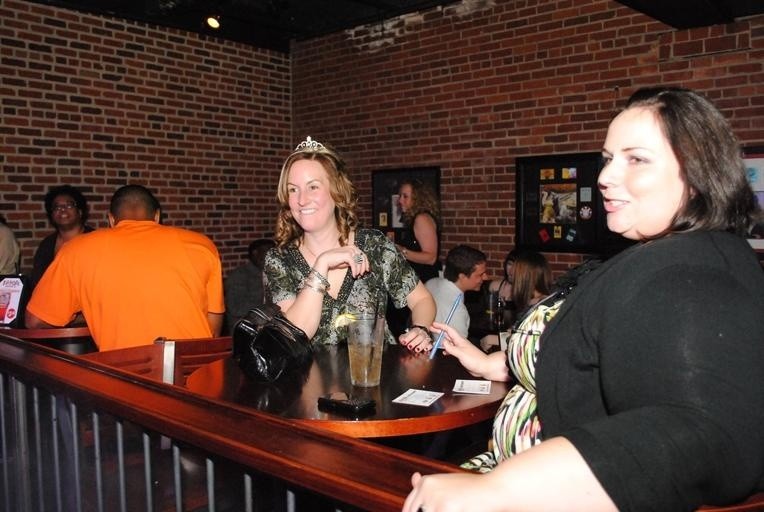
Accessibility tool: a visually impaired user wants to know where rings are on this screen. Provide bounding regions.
[353,255,363,264]
[418,507,423,512]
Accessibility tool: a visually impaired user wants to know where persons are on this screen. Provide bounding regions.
[224,239,276,336]
[486,249,524,311]
[262,135,436,353]
[23,185,226,352]
[401,85,764,512]
[32,185,97,291]
[0,216,21,276]
[394,176,441,283]
[480,252,554,353]
[407,245,488,348]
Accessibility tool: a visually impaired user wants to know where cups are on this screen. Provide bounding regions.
[387,234,394,242]
[346,313,386,388]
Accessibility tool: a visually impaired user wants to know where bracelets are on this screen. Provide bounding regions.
[310,268,330,287]
[410,326,434,341]
[304,280,327,296]
[402,247,407,256]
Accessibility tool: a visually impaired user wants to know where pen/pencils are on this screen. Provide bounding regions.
[428,292,462,360]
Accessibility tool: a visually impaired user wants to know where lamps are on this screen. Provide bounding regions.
[206,13,222,34]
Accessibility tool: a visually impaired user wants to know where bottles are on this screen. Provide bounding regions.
[493,297,505,326]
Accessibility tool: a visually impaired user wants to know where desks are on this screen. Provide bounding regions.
[80,336,508,439]
[0,319,94,355]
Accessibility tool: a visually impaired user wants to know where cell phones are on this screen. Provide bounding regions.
[318,391,377,415]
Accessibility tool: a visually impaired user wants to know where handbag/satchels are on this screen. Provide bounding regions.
[232,303,313,381]
